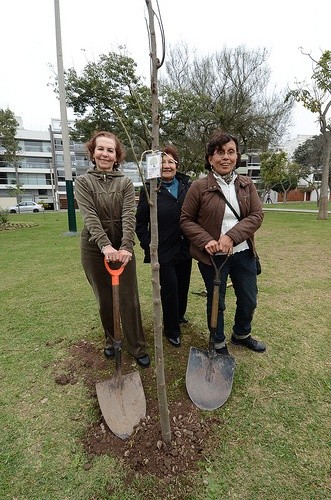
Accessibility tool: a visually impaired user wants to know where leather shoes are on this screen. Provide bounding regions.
[167,336,181,347]
[178,316,188,323]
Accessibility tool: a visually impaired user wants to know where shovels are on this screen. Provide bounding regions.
[186,245,235,411]
[96,252,146,439]
[192,280,233,297]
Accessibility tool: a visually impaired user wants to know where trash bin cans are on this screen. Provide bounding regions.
[43,203,54,210]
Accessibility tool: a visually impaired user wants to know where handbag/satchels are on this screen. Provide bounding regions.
[255,254,261,275]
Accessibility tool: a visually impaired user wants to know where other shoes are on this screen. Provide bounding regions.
[137,352,150,367]
[104,347,116,359]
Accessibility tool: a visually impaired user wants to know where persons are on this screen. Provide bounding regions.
[135,147,195,348]
[180,132,266,355]
[75,132,150,368]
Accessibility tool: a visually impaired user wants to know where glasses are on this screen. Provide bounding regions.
[214,151,237,158]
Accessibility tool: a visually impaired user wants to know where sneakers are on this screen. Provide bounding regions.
[217,344,230,356]
[231,334,266,352]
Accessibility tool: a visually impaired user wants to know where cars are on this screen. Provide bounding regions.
[10,200,45,214]
